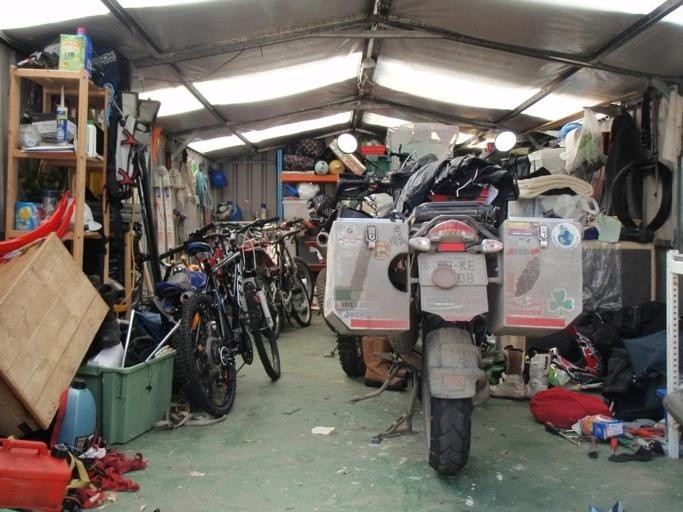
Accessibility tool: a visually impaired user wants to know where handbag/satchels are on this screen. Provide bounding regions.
[556,301,667,362]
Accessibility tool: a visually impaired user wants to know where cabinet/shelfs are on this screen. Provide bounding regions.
[4,61,110,287]
[274,148,363,224]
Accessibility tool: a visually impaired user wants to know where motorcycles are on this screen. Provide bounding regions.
[324,176,585,473]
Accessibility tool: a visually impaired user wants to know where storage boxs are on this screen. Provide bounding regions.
[76,346,178,447]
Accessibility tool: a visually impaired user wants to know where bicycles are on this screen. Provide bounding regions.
[153,217,316,419]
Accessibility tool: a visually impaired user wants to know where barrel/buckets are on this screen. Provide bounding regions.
[58,376,98,453]
[0,439,70,511]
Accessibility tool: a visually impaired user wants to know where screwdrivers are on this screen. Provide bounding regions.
[546,422,578,446]
[611,439,617,456]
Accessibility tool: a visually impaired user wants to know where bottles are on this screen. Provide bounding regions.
[76,27,93,80]
[260,204,267,219]
[86,120,96,157]
[42,190,57,218]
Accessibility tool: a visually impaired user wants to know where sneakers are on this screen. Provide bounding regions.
[488,374,524,399]
[523,379,548,399]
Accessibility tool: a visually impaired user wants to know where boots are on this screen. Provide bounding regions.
[384,338,408,379]
[96,284,120,347]
[361,336,407,390]
